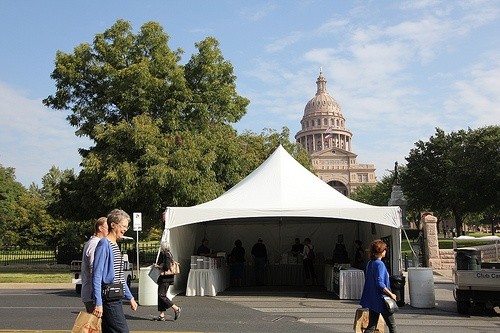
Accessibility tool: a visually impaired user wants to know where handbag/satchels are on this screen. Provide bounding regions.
[101,283,124,301]
[353,308,384,333]
[71,311,102,333]
[149,266,161,284]
[383,296,399,313]
[159,252,180,276]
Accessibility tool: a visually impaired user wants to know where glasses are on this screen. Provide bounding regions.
[118,224,129,229]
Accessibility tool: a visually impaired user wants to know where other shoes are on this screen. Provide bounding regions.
[153,316,165,322]
[174,306,183,321]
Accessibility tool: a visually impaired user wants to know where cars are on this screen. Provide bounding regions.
[54,244,80,264]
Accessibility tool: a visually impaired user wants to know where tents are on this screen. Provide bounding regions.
[163,145,417,306]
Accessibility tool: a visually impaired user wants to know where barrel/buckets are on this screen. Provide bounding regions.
[407,267,435,308]
[138,267,158,306]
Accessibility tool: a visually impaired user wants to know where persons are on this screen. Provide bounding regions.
[93,209,139,333]
[153,240,183,321]
[443,226,456,239]
[196,236,398,333]
[81,217,109,315]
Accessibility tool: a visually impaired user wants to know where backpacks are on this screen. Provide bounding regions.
[306,244,316,264]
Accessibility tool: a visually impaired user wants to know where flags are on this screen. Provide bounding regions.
[325,126,332,140]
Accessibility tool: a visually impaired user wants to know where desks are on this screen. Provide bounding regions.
[325,264,365,300]
[186,266,231,297]
[242,263,324,286]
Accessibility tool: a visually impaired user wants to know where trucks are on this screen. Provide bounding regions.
[451,235,500,317]
